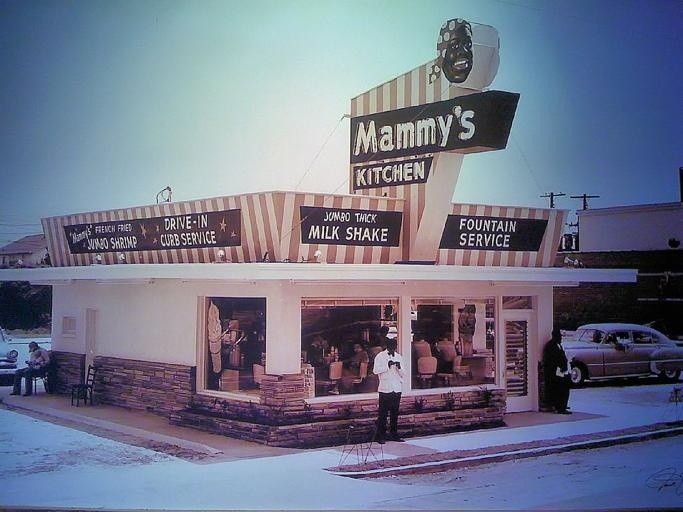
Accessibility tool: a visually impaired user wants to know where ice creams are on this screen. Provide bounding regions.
[208,301,221,373]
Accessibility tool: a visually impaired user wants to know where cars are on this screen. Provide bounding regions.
[0,327,18,385]
[484,315,682,382]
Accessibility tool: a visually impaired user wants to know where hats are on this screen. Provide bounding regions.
[552,330,563,337]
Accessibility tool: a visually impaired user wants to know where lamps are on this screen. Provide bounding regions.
[562,256,585,270]
[213,246,323,263]
[89,252,128,266]
[13,259,22,269]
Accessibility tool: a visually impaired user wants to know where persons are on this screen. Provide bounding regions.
[371,338,405,444]
[234,317,457,395]
[9,340,50,397]
[542,328,573,415]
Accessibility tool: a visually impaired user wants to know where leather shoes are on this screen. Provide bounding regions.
[374,437,385,444]
[557,409,572,414]
[388,435,405,442]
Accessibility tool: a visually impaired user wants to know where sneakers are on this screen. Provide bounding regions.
[10,392,21,396]
[22,391,33,396]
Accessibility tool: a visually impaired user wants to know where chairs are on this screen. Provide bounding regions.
[26,356,54,395]
[71,365,99,407]
[205,332,474,397]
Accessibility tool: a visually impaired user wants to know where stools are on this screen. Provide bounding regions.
[334,419,385,471]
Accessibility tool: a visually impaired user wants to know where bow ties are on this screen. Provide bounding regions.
[388,352,394,357]
